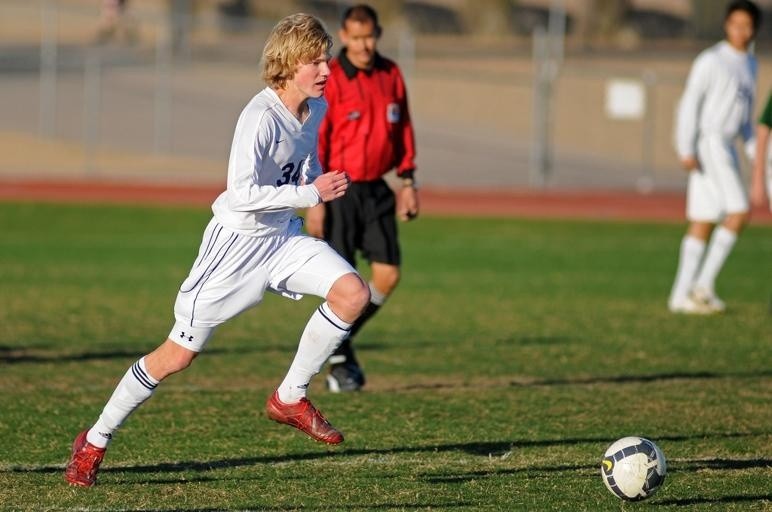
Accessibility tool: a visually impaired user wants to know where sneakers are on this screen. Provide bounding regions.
[264,388,345,445]
[64,428,110,487]
[666,288,726,316]
[322,336,367,395]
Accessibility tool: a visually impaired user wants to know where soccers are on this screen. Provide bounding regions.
[602,437,666,502]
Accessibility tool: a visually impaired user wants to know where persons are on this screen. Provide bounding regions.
[668,1,762,317]
[749,85,772,219]
[66,11,370,491]
[300,3,418,395]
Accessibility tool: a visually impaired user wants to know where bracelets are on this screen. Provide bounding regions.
[402,180,418,191]
